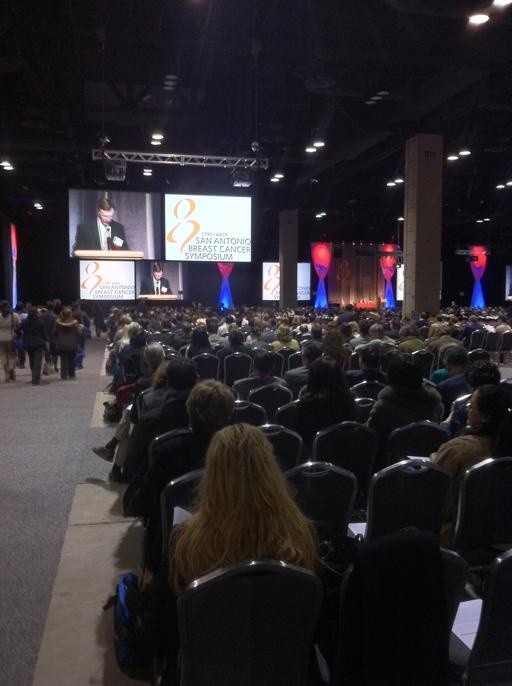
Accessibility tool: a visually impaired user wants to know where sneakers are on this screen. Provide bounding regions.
[109,470,121,481]
[92,446,115,462]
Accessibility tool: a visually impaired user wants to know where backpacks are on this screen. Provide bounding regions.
[112,571,155,682]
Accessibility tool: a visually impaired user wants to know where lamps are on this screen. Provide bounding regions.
[378,242,399,309]
[468,245,488,309]
[216,263,235,310]
[309,242,333,309]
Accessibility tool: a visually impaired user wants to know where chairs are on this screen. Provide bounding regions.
[324,546,467,684]
[154,560,323,685]
[467,328,512,368]
[450,549,512,686]
[136,340,512,557]
[418,325,429,340]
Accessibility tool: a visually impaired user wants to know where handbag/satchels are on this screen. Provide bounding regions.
[70,348,85,368]
[43,352,55,376]
[103,402,122,422]
[110,367,124,392]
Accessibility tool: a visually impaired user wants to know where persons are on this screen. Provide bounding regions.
[73,198,128,252]
[140,263,172,295]
